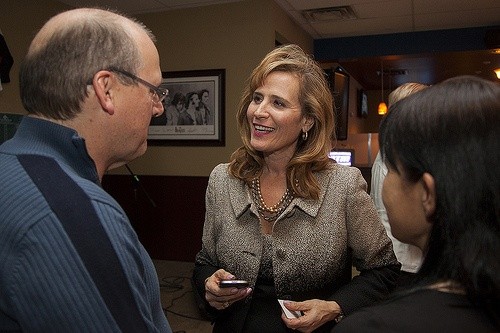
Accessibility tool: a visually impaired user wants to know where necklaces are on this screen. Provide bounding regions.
[250,164,302,223]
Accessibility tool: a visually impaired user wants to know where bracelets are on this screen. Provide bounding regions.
[334,310,344,323]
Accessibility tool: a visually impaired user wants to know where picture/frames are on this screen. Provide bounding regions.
[146,68,226,147]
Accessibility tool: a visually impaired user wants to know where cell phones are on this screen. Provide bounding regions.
[218,280,249,289]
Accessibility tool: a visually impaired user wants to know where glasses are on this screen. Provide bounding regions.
[86,64,170,105]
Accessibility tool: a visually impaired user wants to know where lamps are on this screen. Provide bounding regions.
[378,58,388,115]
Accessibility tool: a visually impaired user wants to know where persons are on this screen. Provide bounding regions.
[0,9,174,333]
[328,75,500,332]
[189,45,399,333]
[370,81,444,279]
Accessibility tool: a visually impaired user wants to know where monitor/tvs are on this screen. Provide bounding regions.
[358,89,368,118]
[328,148,355,168]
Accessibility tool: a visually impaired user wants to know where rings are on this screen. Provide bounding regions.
[224,302,229,307]
[292,328,295,330]
